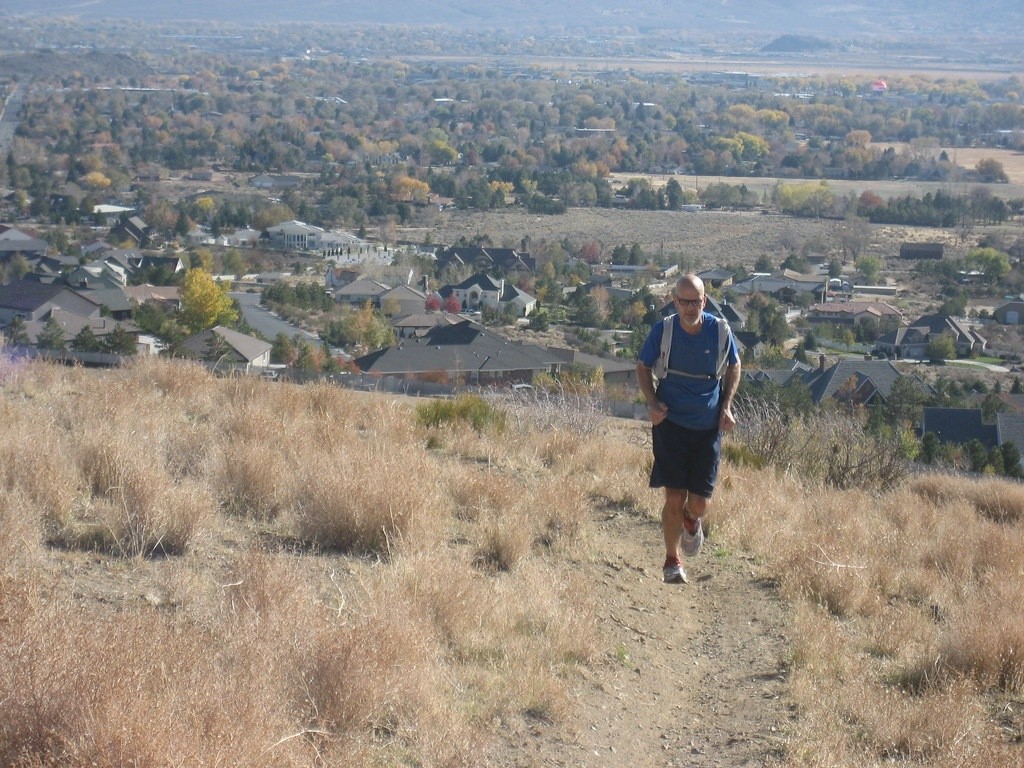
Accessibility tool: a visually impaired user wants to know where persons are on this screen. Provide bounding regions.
[637,275,741,584]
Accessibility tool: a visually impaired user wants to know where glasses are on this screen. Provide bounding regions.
[677,298,703,307]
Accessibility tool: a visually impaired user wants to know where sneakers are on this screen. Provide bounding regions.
[682,500,706,557]
[663,563,688,584]
